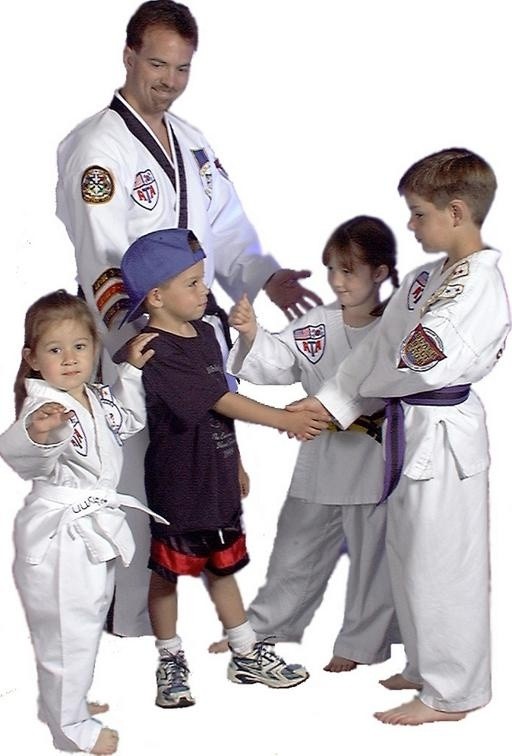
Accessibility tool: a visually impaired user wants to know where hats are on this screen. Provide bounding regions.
[119,228,207,331]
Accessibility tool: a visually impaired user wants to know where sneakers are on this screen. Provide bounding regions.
[228,636,309,688]
[155,647,195,708]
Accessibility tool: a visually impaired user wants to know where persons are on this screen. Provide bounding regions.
[207,214,406,672]
[1,287,162,756]
[118,225,332,711]
[279,146,511,731]
[54,0,324,641]
